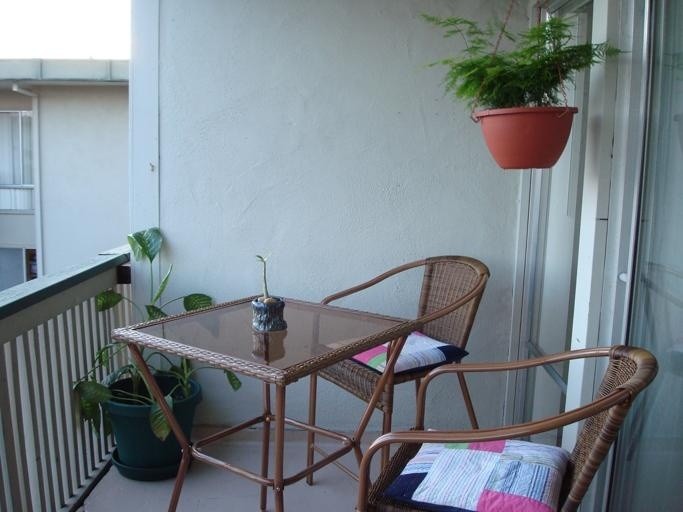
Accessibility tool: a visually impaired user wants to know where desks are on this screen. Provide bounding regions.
[112,290,419,512]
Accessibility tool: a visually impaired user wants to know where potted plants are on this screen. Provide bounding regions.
[70,226,238,483]
[419,2,629,168]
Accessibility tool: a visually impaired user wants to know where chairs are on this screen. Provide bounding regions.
[305,256,490,492]
[357,346,660,512]
